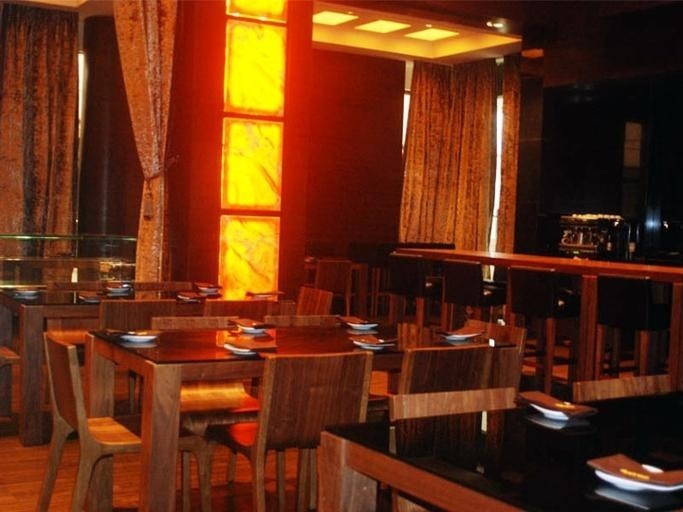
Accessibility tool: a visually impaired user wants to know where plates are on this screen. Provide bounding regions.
[353,339,395,350]
[224,343,269,356]
[347,323,378,330]
[14,291,40,296]
[177,287,219,303]
[441,333,481,340]
[78,287,130,304]
[595,463,683,494]
[238,325,266,334]
[529,399,580,421]
[121,336,158,343]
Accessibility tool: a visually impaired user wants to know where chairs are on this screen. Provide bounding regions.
[572,375,671,404]
[206,351,374,512]
[1,251,526,480]
[35,332,211,511]
[389,386,515,421]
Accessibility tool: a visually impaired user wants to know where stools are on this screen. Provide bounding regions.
[508,265,584,392]
[592,272,670,382]
